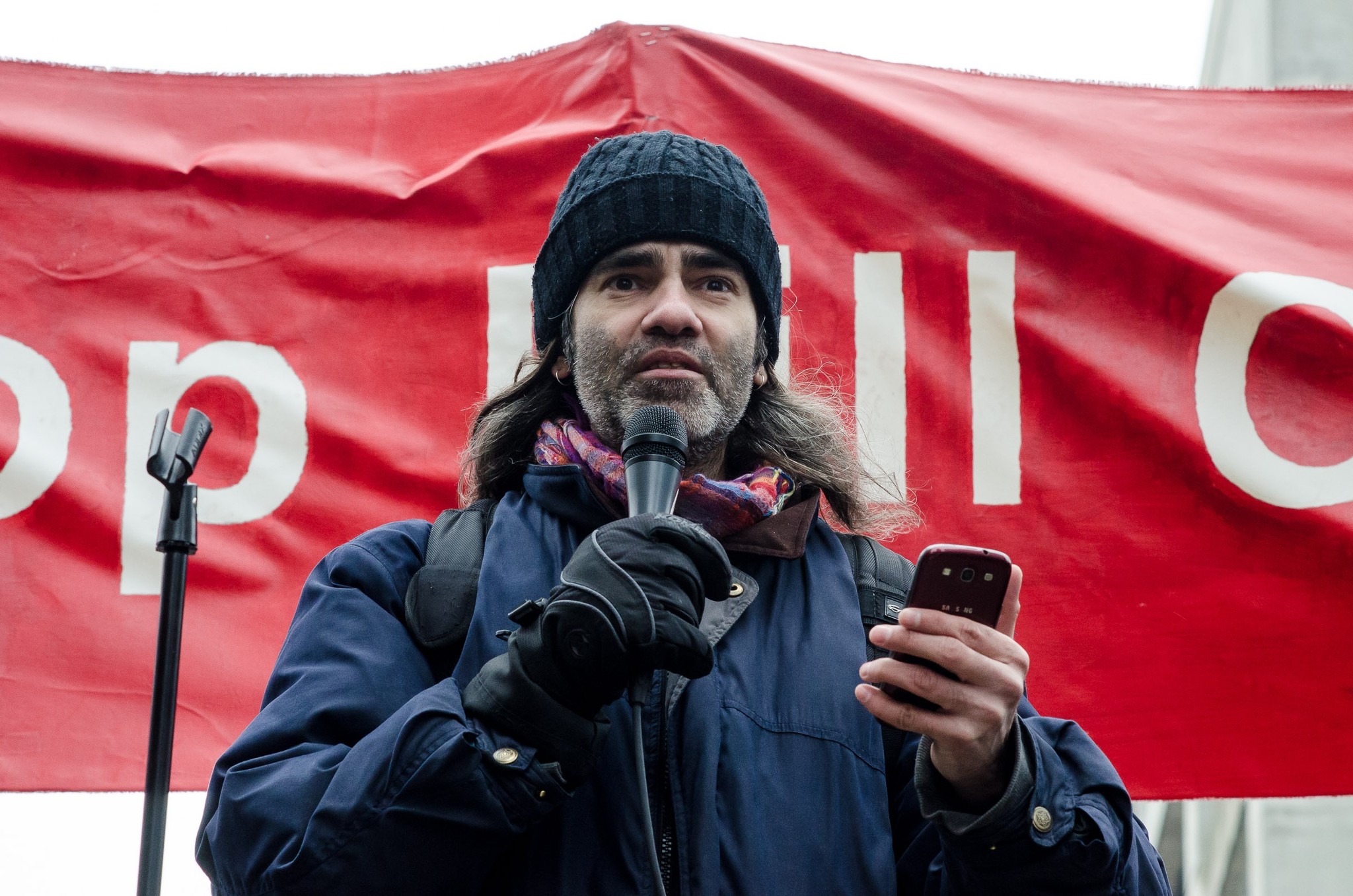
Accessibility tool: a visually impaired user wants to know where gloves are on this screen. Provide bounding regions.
[511,512,735,718]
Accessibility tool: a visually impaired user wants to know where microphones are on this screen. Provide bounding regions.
[619,404,690,710]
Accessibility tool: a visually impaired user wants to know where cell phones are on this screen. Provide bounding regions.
[873,544,1012,734]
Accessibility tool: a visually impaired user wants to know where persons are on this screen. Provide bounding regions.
[195,132,1173,896]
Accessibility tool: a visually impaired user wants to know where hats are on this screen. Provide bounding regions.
[533,130,780,371]
